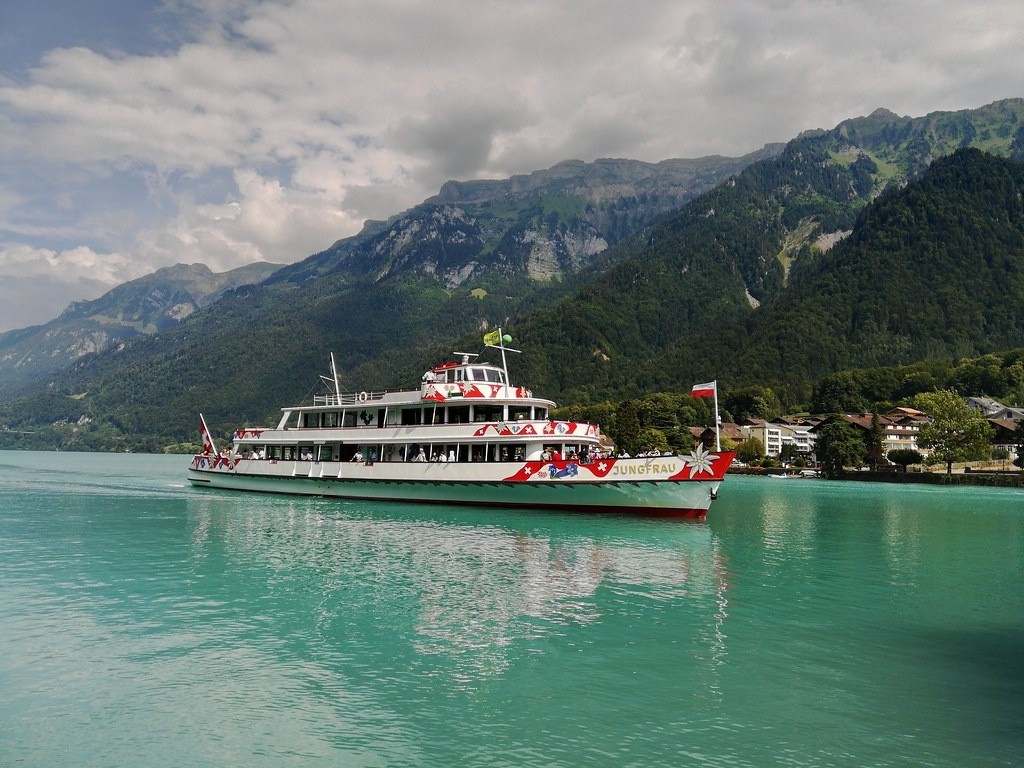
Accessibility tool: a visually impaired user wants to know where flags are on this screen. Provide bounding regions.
[690,382,714,398]
[483,330,500,346]
[199,416,213,452]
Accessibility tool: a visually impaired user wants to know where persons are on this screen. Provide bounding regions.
[577,446,676,464]
[349,450,364,462]
[371,448,377,461]
[413,448,482,463]
[221,446,264,460]
[233,428,238,439]
[288,451,313,461]
[540,448,562,462]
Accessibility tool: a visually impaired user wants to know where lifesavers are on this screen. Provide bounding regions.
[359,392,368,403]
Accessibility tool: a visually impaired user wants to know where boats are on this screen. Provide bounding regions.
[767,472,801,478]
[186,328,738,520]
